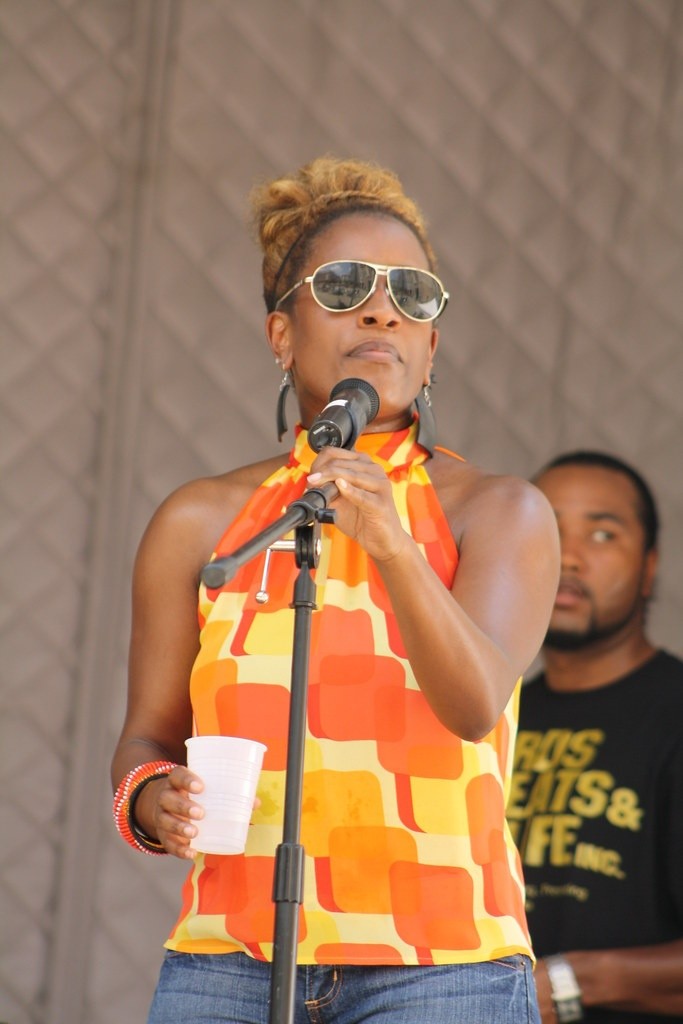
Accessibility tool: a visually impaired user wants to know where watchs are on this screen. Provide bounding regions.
[543,952,583,1024]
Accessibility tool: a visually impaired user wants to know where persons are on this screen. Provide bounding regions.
[111,156,561,1022]
[512,449,683,1023]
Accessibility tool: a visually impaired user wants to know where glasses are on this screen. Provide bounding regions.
[270,260,450,323]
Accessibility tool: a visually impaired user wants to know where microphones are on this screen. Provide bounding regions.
[308,378,380,455]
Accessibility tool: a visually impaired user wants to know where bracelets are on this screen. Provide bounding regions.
[113,761,183,861]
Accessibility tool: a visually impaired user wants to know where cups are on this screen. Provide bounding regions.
[183,735,268,856]
